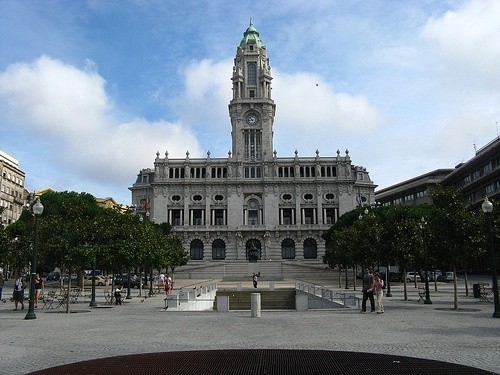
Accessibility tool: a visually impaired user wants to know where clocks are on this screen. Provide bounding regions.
[247,111,260,126]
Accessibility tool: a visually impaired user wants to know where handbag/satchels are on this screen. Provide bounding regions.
[379,279,386,290]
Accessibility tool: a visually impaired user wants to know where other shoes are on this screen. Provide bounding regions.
[370,310,376,313]
[35,306,37,308]
[21,308,24,310]
[360,309,367,313]
[13,309,18,311]
[376,311,384,315]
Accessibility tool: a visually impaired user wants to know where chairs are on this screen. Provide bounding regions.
[103,289,126,307]
[417,285,429,301]
[478,281,495,304]
[42,288,81,309]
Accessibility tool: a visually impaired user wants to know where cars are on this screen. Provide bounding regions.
[356,269,458,283]
[20,273,30,282]
[46,271,60,281]
[58,269,175,290]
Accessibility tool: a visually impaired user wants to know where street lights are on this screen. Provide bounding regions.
[482,196,500,318]
[420,216,433,304]
[139,210,156,289]
[24,198,44,320]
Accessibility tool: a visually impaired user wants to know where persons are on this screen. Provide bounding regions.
[34,273,44,308]
[360,266,375,313]
[160,272,173,295]
[12,274,25,311]
[0,273,4,300]
[105,274,109,286]
[253,273,260,288]
[369,272,384,314]
